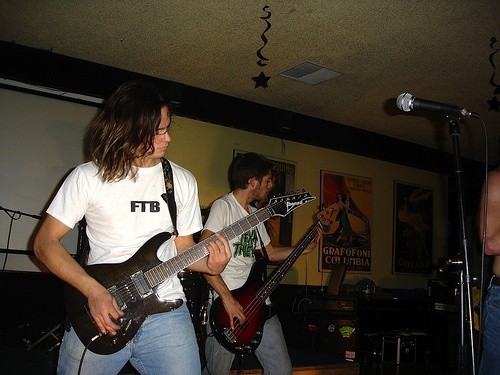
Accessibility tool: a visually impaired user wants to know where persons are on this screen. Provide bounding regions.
[196,151,325,375]
[30,80,233,375]
[476,165,500,375]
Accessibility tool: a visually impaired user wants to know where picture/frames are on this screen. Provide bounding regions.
[319,169,372,275]
[391,179,437,278]
[233,149,299,250]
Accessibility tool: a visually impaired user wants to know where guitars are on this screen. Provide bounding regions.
[68,188,318,355]
[209,202,344,355]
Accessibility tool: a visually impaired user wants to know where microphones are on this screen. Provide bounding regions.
[396,93,479,118]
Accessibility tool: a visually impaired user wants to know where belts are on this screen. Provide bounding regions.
[492,276,500,286]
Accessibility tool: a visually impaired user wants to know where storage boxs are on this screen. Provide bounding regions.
[380,334,417,366]
